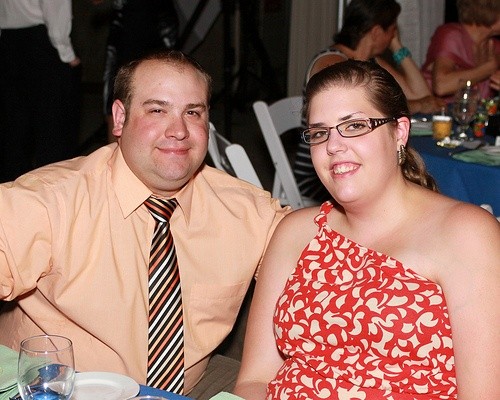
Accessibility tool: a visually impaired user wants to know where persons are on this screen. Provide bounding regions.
[0,0,83,171]
[233,0,500,400]
[0,51,290,400]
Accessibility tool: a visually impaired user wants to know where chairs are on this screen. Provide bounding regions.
[207,121,263,197]
[253,95,319,212]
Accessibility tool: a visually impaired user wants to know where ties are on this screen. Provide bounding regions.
[142,196,186,395]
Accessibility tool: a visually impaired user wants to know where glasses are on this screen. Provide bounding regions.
[300,116,398,145]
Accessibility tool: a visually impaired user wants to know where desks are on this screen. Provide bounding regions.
[409,112,500,217]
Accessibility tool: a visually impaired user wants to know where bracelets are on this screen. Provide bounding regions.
[393,47,411,63]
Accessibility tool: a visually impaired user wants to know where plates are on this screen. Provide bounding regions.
[68,372,140,400]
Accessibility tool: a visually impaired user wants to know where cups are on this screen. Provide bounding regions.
[431,116,454,142]
[17,334,76,400]
